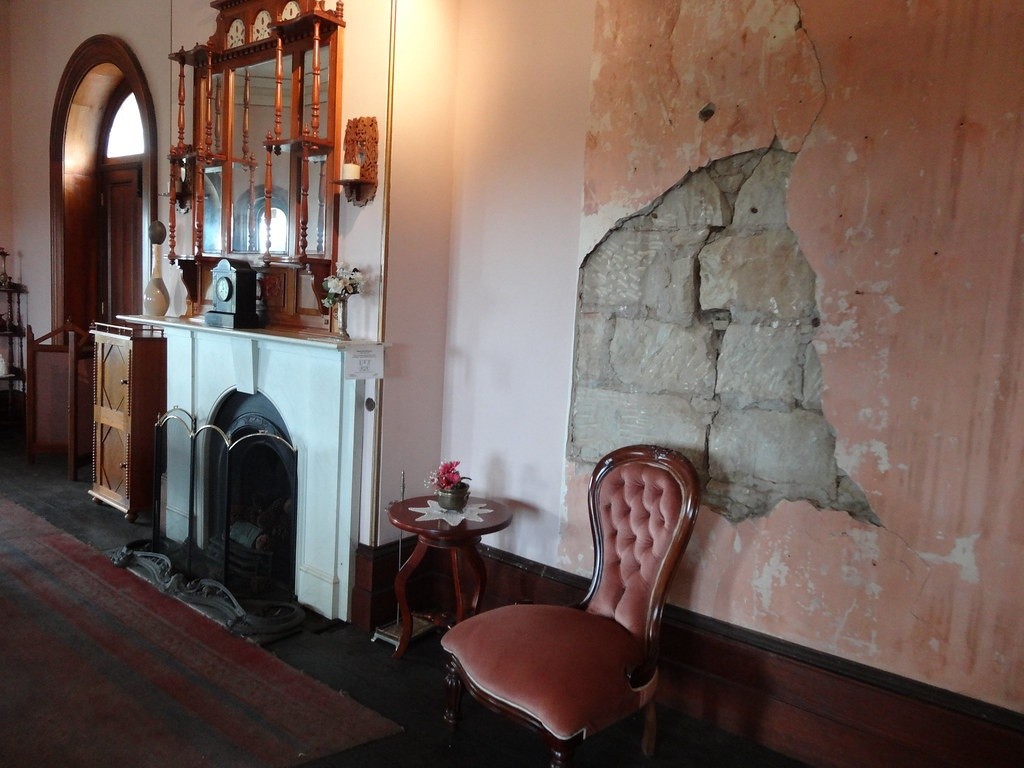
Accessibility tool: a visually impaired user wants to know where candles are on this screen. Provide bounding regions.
[341,160,360,180]
[167,180,181,193]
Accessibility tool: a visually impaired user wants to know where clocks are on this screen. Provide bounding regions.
[204,257,258,331]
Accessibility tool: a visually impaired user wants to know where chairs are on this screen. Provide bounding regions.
[438,443,703,768]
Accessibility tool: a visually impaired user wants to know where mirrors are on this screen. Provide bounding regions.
[204,46,326,258]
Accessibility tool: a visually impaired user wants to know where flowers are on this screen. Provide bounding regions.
[321,260,370,309]
[422,458,472,496]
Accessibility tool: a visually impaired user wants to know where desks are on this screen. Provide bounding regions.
[0,496,408,768]
[387,496,512,660]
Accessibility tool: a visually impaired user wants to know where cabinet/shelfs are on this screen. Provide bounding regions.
[0,286,26,440]
[87,320,168,523]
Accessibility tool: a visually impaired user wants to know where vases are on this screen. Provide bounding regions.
[436,483,471,514]
[337,301,351,341]
